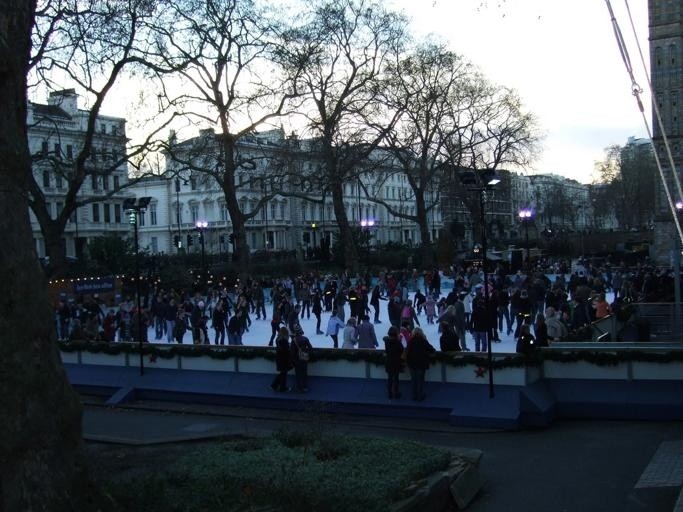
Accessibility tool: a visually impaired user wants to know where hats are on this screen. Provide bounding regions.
[593,294,601,302]
[387,326,398,336]
[537,313,546,320]
[294,325,304,334]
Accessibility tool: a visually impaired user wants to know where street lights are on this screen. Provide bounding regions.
[172,176,189,249]
[196,222,208,266]
[459,176,502,400]
[360,219,375,255]
[471,244,479,260]
[310,222,318,257]
[121,194,153,379]
[517,209,534,273]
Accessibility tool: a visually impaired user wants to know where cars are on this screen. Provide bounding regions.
[39,254,78,269]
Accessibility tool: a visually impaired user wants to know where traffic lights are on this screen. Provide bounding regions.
[228,233,233,244]
[186,234,194,248]
[172,234,178,248]
[220,235,225,243]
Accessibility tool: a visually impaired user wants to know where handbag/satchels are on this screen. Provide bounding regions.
[299,351,309,360]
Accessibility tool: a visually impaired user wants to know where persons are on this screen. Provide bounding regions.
[42,225,683,401]
